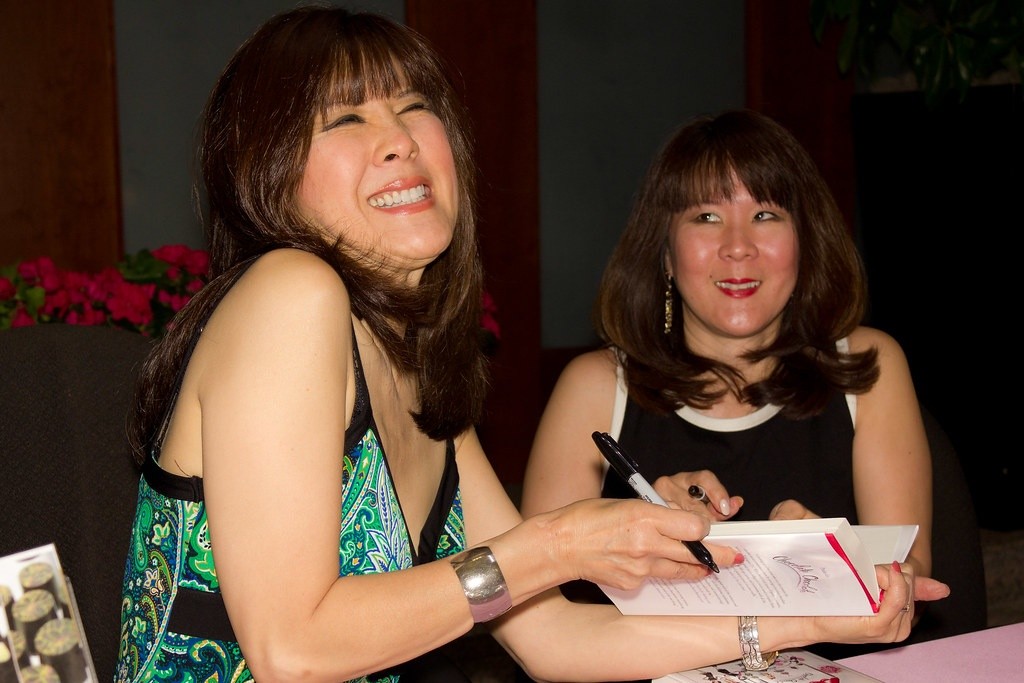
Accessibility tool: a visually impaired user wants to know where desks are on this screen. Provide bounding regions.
[834,620,1024,682]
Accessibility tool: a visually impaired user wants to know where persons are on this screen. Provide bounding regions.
[114,5,950,683]
[517,108,934,662]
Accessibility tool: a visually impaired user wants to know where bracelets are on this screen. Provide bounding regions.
[448,545,513,625]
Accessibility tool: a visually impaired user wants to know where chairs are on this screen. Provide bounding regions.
[914,406,988,644]
[0,321,160,683]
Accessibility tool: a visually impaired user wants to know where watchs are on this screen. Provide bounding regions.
[738,616,779,672]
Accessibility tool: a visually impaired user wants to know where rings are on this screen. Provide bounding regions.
[902,604,911,613]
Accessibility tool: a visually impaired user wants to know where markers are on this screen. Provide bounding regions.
[686,480,712,507]
[591,430,721,577]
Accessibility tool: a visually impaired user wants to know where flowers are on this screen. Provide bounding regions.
[0,241,505,338]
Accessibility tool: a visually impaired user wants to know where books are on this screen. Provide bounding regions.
[597,518,920,616]
